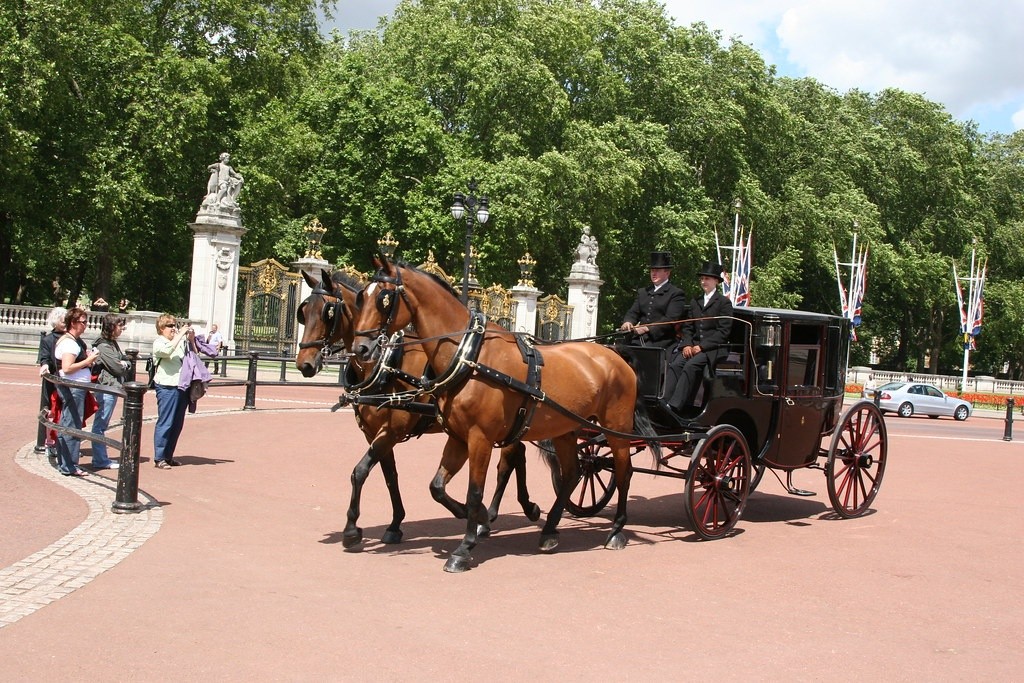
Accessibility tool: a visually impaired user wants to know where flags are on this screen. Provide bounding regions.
[717,238,751,306]
[958,277,983,350]
[839,267,866,341]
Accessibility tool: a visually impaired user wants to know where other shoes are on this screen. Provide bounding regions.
[79,452,83,457]
[155,458,182,469]
[61,466,89,476]
[47,447,57,457]
[656,396,671,411]
[93,460,119,469]
[34,446,45,453]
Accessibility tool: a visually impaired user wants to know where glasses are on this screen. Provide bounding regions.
[73,320,88,324]
[164,324,176,327]
[116,323,125,326]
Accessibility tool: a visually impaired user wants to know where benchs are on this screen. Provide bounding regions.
[611,336,779,407]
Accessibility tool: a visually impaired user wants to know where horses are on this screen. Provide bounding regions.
[293,248,664,574]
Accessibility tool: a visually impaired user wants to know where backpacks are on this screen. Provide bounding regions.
[145,356,163,390]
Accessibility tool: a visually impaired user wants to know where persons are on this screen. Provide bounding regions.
[53,308,100,476]
[908,377,913,382]
[203,324,222,374]
[118,298,128,326]
[91,313,132,468]
[619,251,686,348]
[863,375,876,399]
[39,307,69,456]
[153,313,199,469]
[661,261,733,411]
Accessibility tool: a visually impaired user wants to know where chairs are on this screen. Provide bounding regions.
[917,387,922,393]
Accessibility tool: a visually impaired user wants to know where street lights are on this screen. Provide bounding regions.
[451,193,491,307]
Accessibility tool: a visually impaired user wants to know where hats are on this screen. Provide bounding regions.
[696,261,724,284]
[647,252,674,268]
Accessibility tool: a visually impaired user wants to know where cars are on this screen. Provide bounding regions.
[875,382,974,420]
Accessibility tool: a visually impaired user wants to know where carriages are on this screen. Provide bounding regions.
[295,252,887,571]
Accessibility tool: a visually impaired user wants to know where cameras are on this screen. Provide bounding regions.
[91,347,99,355]
[185,328,194,335]
